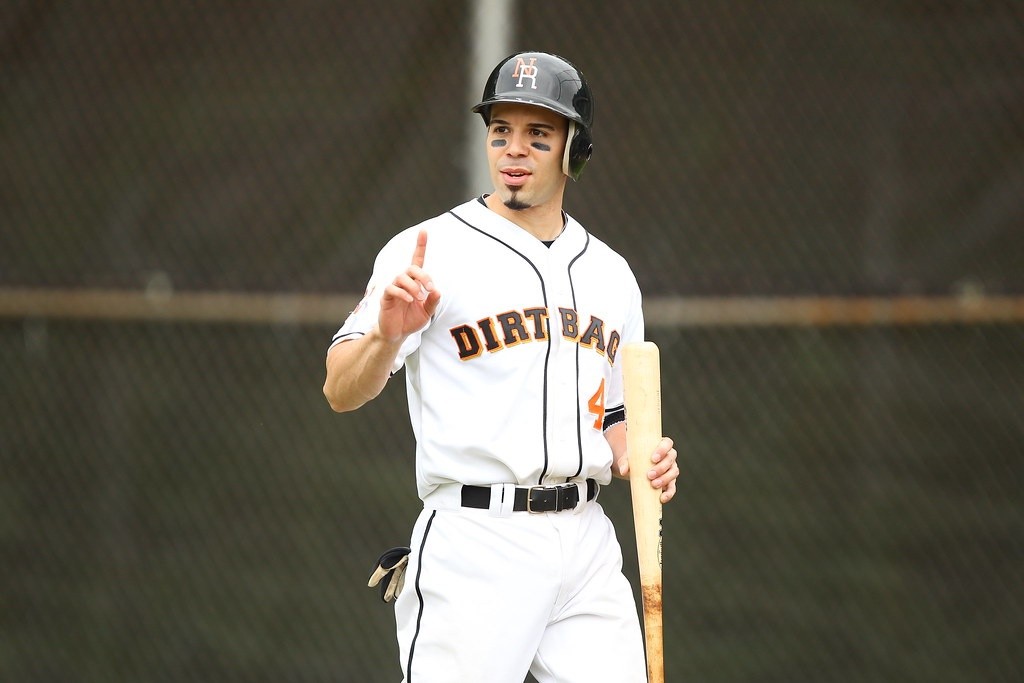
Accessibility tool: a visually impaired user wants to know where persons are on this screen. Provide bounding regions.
[323,50,679,683]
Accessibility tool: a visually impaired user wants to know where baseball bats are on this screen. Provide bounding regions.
[619,340,680,683]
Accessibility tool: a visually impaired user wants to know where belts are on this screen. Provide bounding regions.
[461,478,597,514]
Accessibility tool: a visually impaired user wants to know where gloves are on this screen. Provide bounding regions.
[366,546,411,604]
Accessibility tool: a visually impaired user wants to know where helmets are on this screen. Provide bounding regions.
[470,50,595,183]
